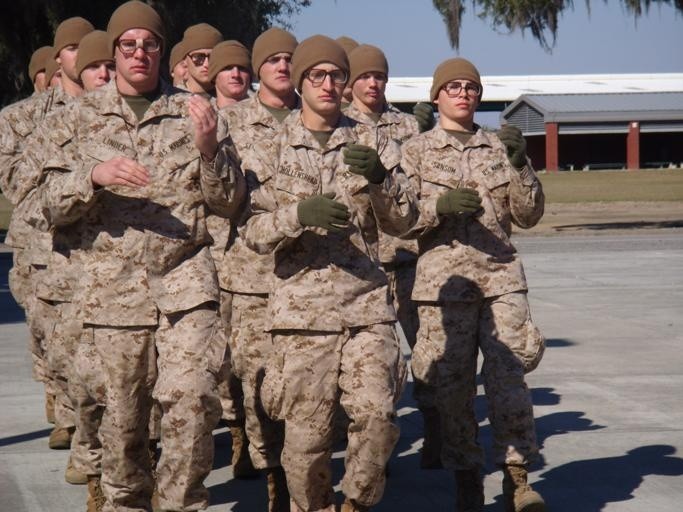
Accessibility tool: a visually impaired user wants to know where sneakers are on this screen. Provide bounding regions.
[47,404,57,424]
[86,476,106,512]
[454,470,484,511]
[65,461,89,484]
[49,427,70,449]
[502,464,545,511]
[232,424,261,479]
[420,446,443,470]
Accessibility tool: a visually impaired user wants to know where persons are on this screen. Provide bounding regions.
[398,54,553,512]
[30,33,118,488]
[330,36,364,58]
[204,38,253,110]
[167,41,194,90]
[41,0,249,512]
[174,19,230,93]
[212,26,332,481]
[5,16,100,453]
[345,46,448,467]
[0,45,50,247]
[242,35,422,512]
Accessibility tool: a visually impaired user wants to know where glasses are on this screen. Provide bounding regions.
[115,38,161,54]
[187,52,210,66]
[304,69,348,85]
[441,82,483,99]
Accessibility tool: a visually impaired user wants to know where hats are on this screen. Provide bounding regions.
[430,58,482,102]
[46,51,61,89]
[29,46,53,82]
[170,41,184,72]
[107,1,164,59]
[348,44,388,88]
[75,30,115,80]
[335,37,359,53]
[54,17,94,59]
[252,28,300,80]
[208,40,252,82]
[290,35,350,95]
[184,23,223,58]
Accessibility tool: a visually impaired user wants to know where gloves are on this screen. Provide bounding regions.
[436,189,484,215]
[343,144,386,183]
[298,190,351,235]
[414,102,436,132]
[497,126,528,168]
